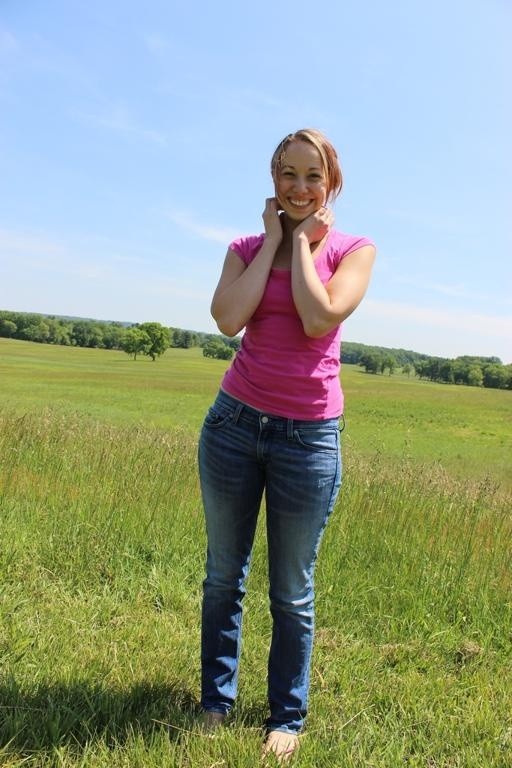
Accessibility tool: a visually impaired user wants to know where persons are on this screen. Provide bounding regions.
[199,128,376,755]
